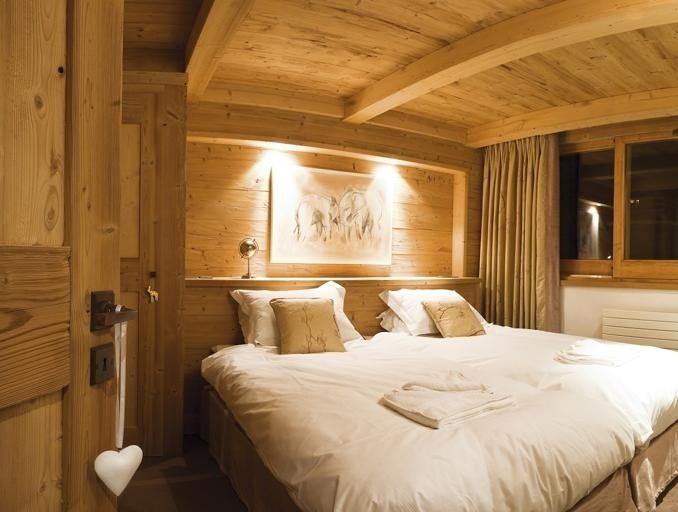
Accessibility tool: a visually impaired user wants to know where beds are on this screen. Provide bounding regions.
[200,323,678,512]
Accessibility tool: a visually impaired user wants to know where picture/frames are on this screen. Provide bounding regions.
[270,165,393,266]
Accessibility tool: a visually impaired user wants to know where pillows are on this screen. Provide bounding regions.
[230,279,490,355]
